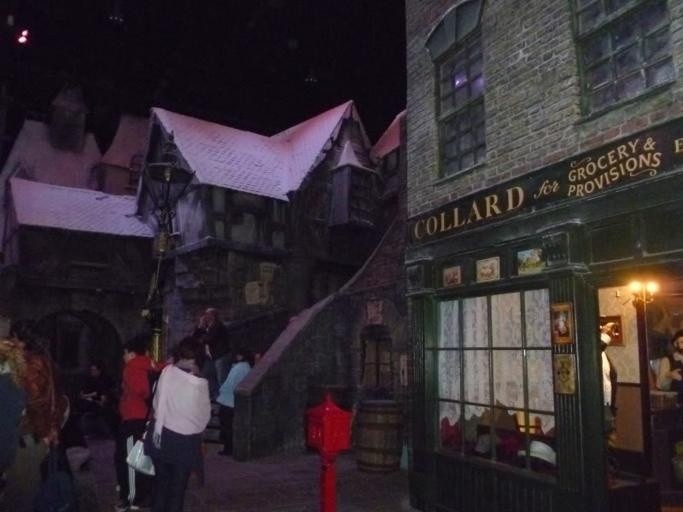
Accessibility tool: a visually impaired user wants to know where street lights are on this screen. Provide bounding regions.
[132,120,196,375]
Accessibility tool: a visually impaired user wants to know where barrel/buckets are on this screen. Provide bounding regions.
[354,398,403,475]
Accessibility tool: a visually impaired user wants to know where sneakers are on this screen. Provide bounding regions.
[114,502,140,511]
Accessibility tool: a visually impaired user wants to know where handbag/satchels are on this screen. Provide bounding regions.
[126,425,160,477]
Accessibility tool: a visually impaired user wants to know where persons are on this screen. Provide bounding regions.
[601,331,619,489]
[656,329,683,409]
[2,306,253,511]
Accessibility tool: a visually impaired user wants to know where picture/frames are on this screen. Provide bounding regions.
[599,314,624,348]
[549,302,575,345]
